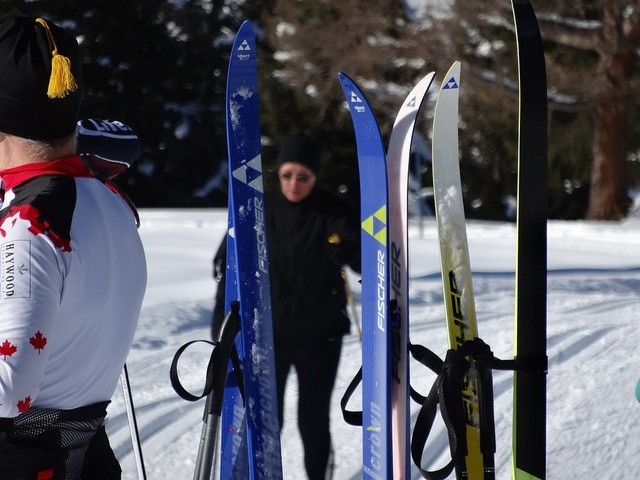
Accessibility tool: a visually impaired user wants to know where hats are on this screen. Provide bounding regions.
[0,17,82,140]
[278,134,319,176]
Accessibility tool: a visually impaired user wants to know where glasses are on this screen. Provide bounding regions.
[279,171,311,185]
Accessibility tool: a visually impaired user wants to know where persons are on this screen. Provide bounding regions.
[212,133,362,479]
[0,17,147,480]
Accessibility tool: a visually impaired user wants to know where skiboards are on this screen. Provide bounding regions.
[220,19,283,480]
[431,0,548,480]
[337,71,436,480]
